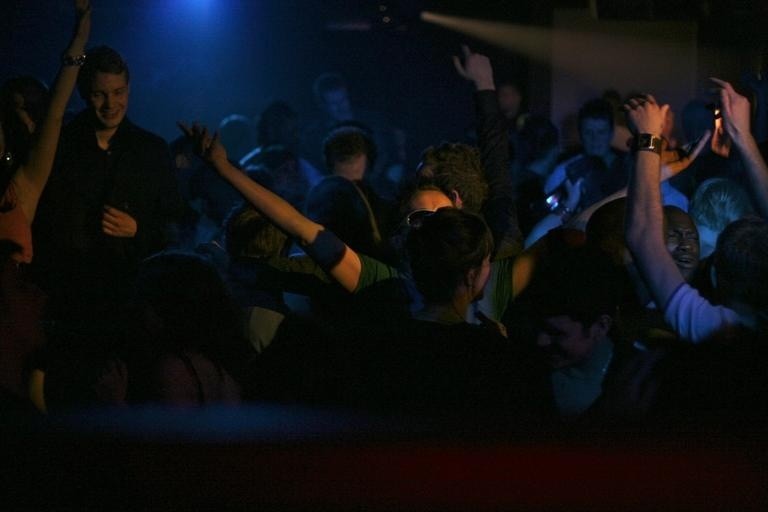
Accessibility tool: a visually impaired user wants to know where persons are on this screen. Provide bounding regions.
[1,1,768,509]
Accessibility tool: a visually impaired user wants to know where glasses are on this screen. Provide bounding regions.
[396,208,450,228]
